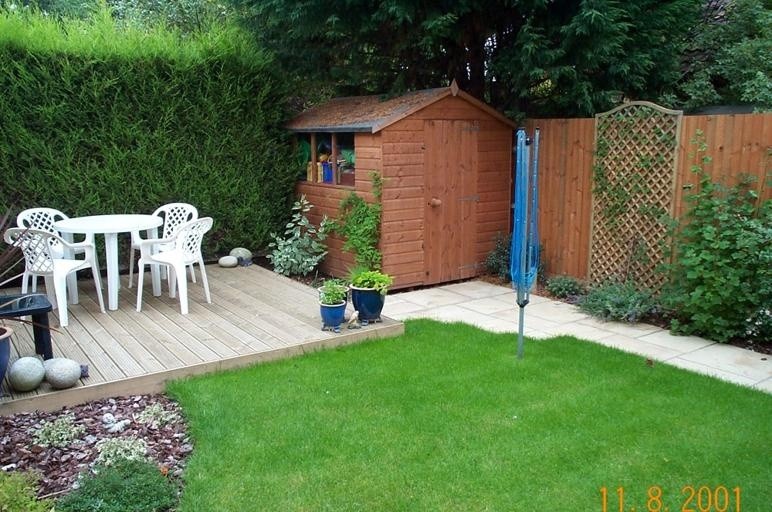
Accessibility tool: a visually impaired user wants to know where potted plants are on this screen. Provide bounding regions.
[317,279,349,333]
[344,271,395,324]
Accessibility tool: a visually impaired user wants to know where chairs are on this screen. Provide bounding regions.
[3,228,106,329]
[17,208,104,306]
[136,217,213,315]
[128,203,198,287]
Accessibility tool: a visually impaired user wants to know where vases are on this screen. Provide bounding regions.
[0,326,13,385]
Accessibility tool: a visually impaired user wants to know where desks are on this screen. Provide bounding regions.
[55,214,163,310]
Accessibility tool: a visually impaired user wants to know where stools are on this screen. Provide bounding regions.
[0,293,53,361]
